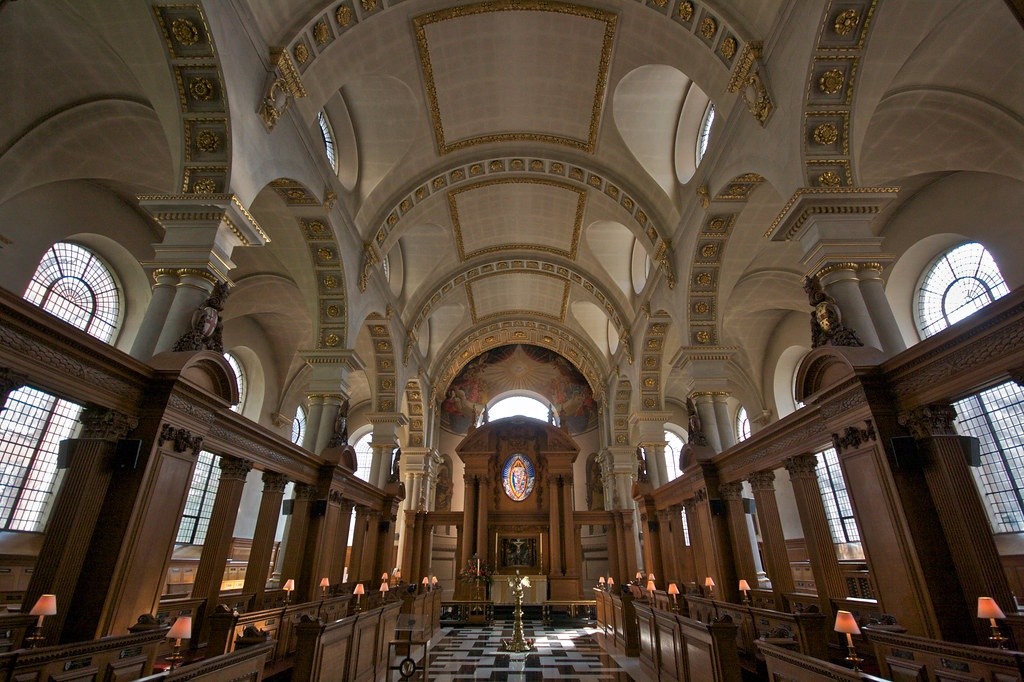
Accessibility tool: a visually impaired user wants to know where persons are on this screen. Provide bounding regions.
[435,464,454,513]
[585,462,606,512]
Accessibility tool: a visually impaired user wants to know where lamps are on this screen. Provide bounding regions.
[283,579,294,606]
[432,576,438,589]
[978,597,1008,650]
[25,594,57,650]
[668,583,681,614]
[353,584,364,614]
[739,580,752,605]
[599,572,656,597]
[394,572,401,584]
[164,617,192,671]
[320,577,329,599]
[833,610,865,671]
[705,577,715,600]
[379,582,389,606]
[381,573,388,582]
[422,577,428,593]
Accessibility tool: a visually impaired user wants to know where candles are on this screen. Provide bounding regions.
[477,559,480,576]
[495,532,498,555]
[540,533,542,554]
